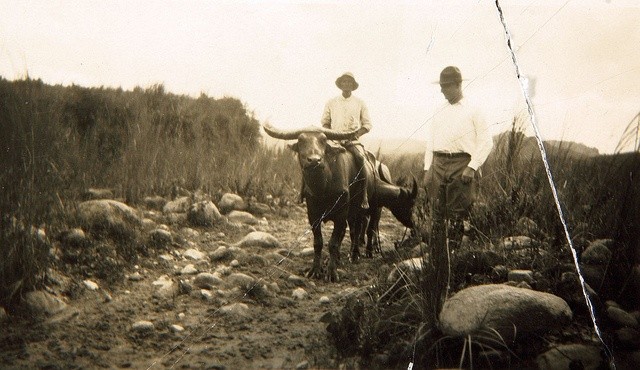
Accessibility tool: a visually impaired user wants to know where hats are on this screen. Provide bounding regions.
[336,70,359,89]
[430,66,470,84]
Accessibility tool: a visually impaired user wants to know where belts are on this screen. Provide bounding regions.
[435,151,470,158]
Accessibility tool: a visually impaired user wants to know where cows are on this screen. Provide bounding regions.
[263,123,377,285]
[355,159,418,259]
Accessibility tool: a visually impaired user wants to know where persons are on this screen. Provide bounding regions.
[423,66,494,218]
[321,72,372,209]
